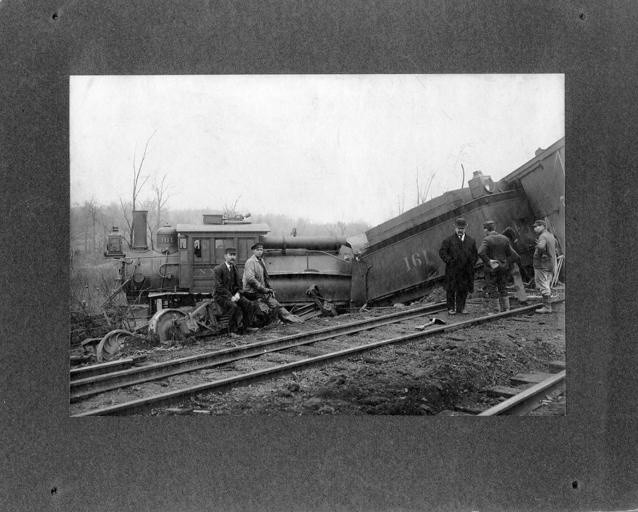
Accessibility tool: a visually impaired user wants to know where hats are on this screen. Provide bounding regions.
[251,242,264,250]
[453,218,468,227]
[483,221,495,226]
[225,248,237,254]
[529,220,546,227]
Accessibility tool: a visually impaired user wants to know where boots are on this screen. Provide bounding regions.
[535,295,552,314]
[488,297,510,316]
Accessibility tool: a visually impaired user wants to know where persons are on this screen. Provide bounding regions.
[214,242,298,338]
[528,220,558,314]
[439,218,530,316]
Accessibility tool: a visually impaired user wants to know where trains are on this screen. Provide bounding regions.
[104,136,565,305]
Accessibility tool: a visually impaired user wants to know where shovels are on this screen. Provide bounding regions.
[415,318,446,331]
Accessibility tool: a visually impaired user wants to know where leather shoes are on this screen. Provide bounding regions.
[448,310,470,314]
[244,328,259,334]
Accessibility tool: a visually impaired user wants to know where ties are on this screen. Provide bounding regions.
[230,263,233,273]
[459,235,462,240]
